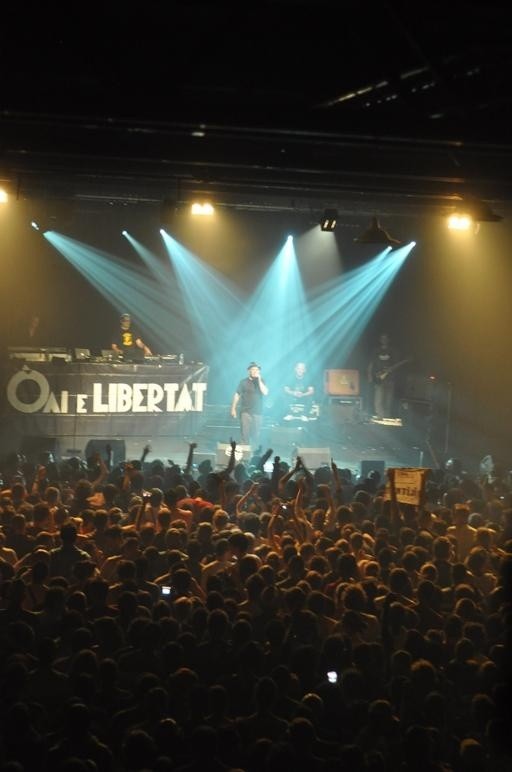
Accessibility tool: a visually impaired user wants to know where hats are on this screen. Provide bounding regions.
[248,362,260,370]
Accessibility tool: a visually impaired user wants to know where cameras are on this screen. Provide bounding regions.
[160,584,173,596]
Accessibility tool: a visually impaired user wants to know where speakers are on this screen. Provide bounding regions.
[291,448,331,469]
[85,439,125,462]
[23,435,60,459]
[216,444,251,466]
[327,369,361,396]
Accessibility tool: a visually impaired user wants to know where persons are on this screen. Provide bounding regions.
[112,313,154,356]
[1,439,510,771]
[366,333,409,421]
[281,361,315,416]
[11,312,48,346]
[229,360,269,443]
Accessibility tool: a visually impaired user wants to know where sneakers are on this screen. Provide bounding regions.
[377,415,382,420]
